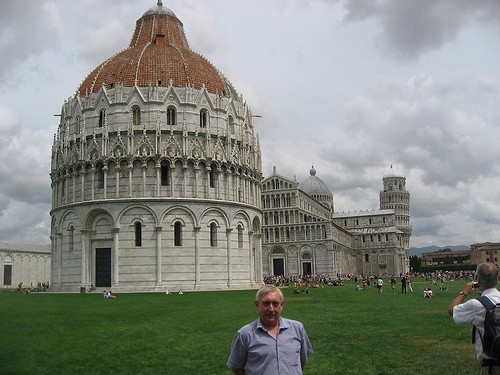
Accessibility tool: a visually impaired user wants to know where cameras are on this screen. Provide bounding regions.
[472,281,480,288]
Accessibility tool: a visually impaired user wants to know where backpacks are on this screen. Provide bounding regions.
[472,295,500,358]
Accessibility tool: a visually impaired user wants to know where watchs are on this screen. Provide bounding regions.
[460,291,467,297]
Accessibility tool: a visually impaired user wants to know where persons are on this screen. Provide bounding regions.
[263,269,482,298]
[447,262,500,375]
[1,281,119,299]
[226,285,314,375]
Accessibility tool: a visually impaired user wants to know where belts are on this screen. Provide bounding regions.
[480,359,497,368]
